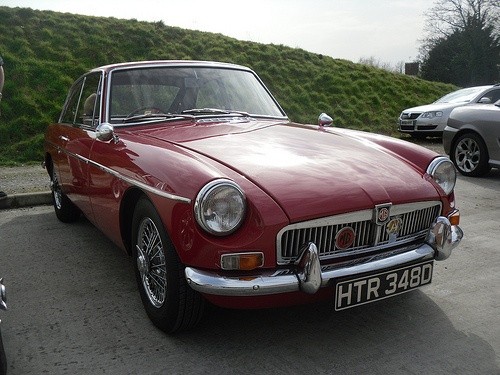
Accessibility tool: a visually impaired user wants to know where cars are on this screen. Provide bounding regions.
[42,58,463,335]
[396,83,500,134]
[443,83,500,174]
